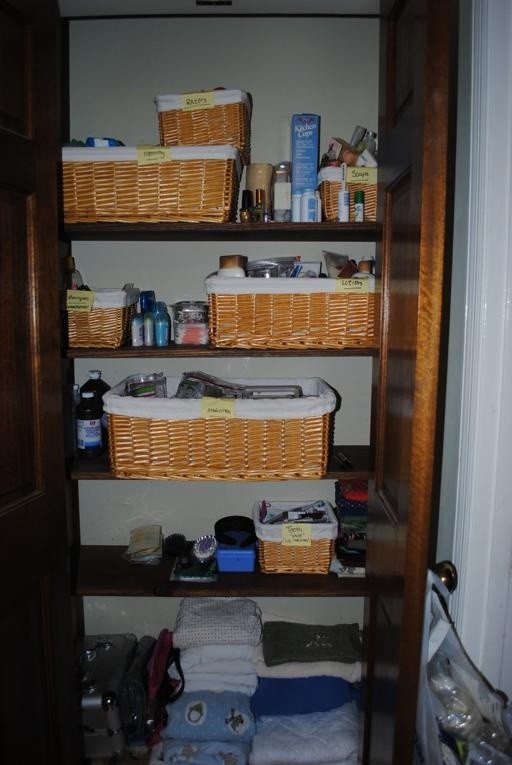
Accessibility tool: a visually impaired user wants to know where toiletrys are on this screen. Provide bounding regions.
[130,290,170,347]
[236,184,366,224]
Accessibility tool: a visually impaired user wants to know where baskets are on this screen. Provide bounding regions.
[153,89,251,165]
[203,268,380,351]
[102,372,339,480]
[65,287,138,349]
[316,165,378,222]
[252,497,339,576]
[61,144,244,225]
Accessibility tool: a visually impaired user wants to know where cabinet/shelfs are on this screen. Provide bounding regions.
[1,0,463,764]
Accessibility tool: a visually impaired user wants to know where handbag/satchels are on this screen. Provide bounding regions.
[118,626,177,750]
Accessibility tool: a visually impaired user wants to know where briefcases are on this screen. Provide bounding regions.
[79,632,139,761]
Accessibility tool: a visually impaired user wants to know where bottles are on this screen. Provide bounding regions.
[360,129,378,152]
[349,261,374,279]
[131,293,169,347]
[354,189,364,222]
[289,186,325,225]
[72,368,107,452]
[215,256,245,278]
